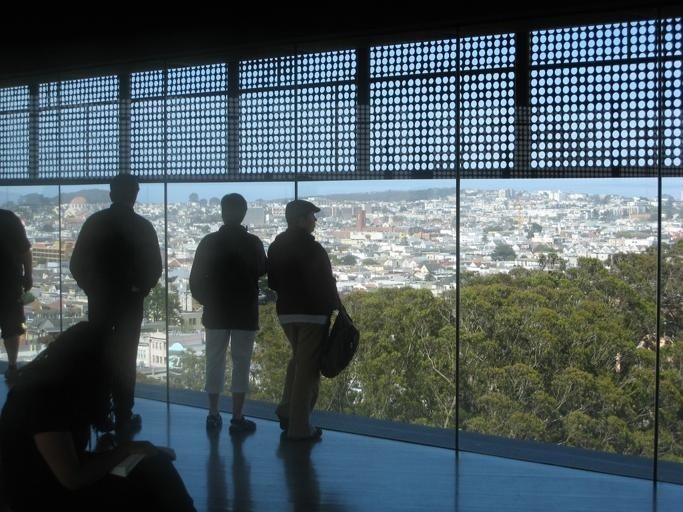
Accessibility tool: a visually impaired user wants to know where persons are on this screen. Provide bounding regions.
[0,205,33,379]
[188,193,269,436]
[0,322,195,512]
[267,199,342,442]
[69,173,164,438]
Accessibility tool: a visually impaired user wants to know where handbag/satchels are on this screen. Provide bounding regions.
[318,310,359,378]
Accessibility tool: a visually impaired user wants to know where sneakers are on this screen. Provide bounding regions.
[95,414,321,442]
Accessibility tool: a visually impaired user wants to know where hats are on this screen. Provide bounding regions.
[286,200,320,217]
